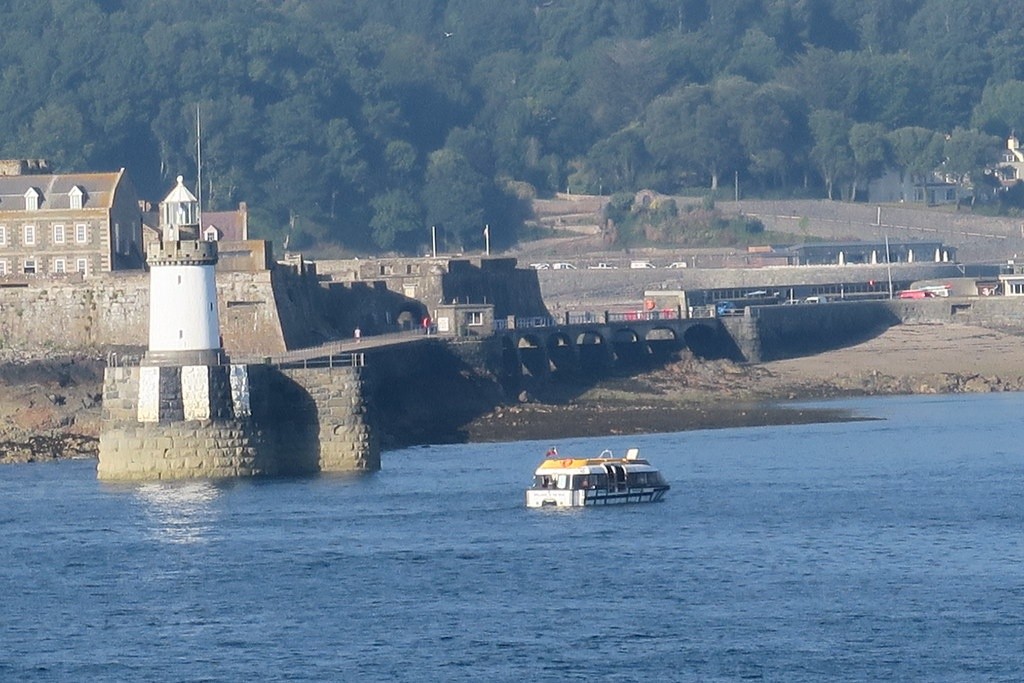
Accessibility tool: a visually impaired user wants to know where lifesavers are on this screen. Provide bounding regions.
[646,299,655,310]
[563,458,573,467]
[424,318,430,328]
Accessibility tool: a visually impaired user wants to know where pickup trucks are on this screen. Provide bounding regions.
[587,262,619,270]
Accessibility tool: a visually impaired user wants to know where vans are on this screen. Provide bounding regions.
[665,261,687,268]
[552,263,576,269]
[631,261,656,268]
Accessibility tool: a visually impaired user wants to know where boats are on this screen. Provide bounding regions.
[525,447,672,509]
